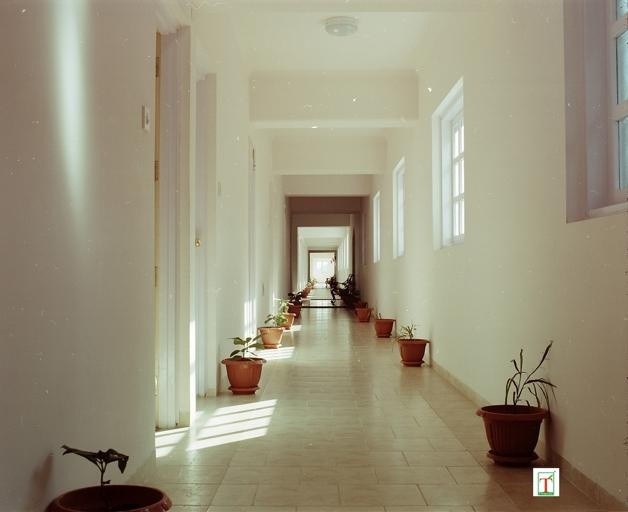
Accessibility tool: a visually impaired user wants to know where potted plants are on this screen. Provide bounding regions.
[475,337,559,466]
[221,277,317,394]
[49,444,173,511]
[347,290,430,368]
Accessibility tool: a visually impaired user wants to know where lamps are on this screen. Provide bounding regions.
[325,17,359,38]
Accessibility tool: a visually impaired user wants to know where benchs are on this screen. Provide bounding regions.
[330,273,353,304]
